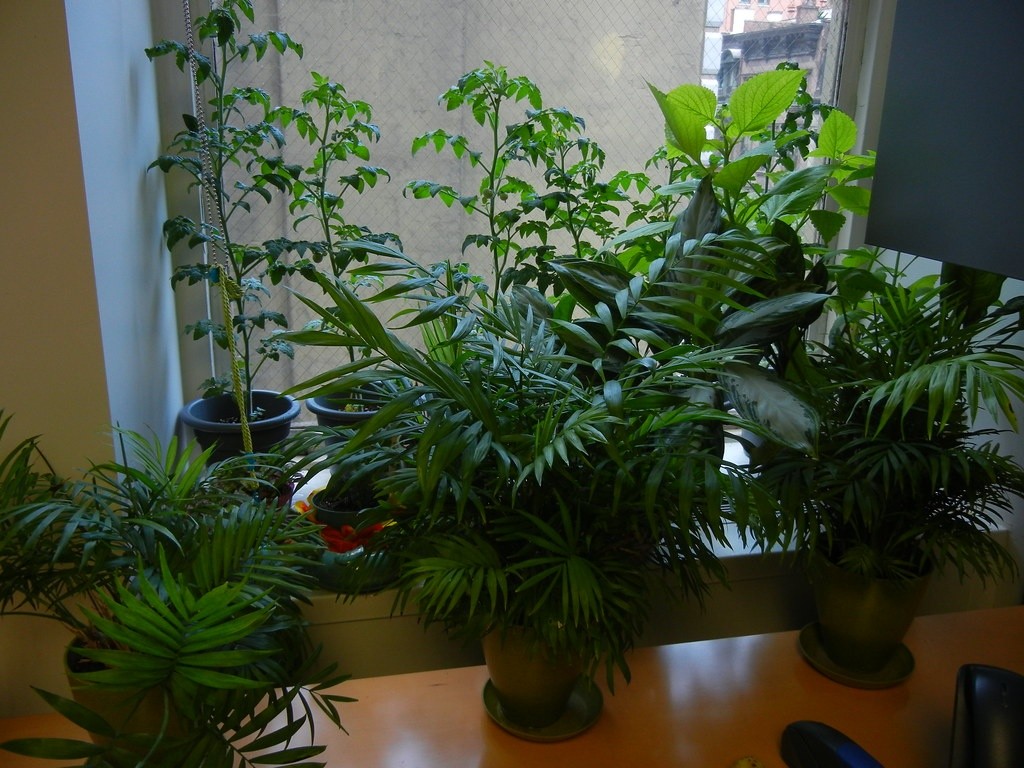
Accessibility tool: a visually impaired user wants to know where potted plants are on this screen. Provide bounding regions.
[0,0,1024,768]
